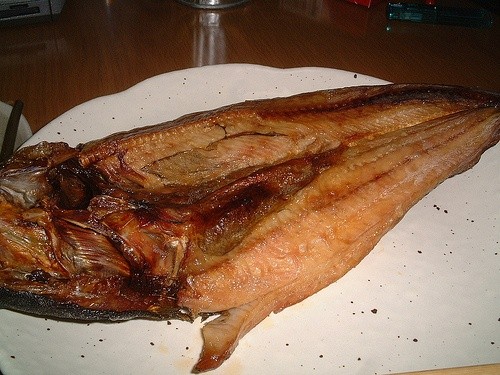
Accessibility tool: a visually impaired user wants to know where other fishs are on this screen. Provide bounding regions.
[1,84,499,375]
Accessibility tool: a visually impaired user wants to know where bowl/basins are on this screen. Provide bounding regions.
[0,101,33,153]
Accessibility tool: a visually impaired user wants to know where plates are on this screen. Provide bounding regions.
[1,63,499,375]
